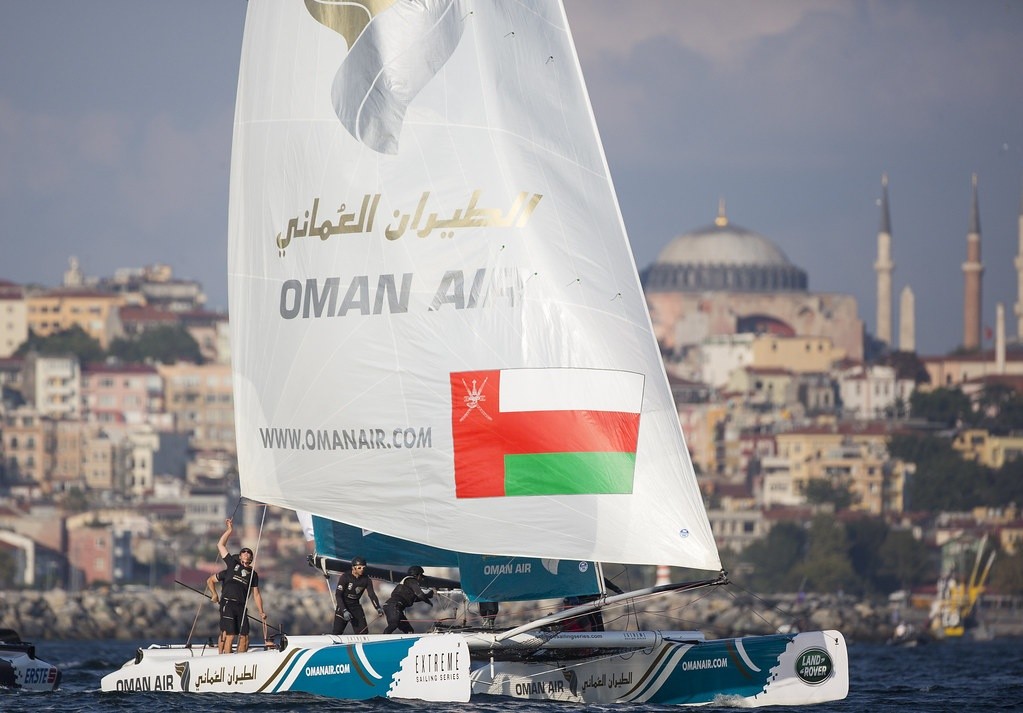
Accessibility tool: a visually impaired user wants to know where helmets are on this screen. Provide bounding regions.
[407,566,424,576]
[351,556,366,566]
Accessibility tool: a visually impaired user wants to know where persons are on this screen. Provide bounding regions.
[577,576,623,631]
[382,564,435,634]
[331,557,384,634]
[547,597,591,632]
[478,602,499,626]
[206,554,250,654]
[215,518,267,654]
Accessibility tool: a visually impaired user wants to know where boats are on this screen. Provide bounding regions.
[1,628,63,691]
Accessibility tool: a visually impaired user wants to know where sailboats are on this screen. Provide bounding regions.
[102,1,850,708]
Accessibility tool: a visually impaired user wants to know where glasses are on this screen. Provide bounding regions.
[354,566,366,569]
[240,550,252,554]
